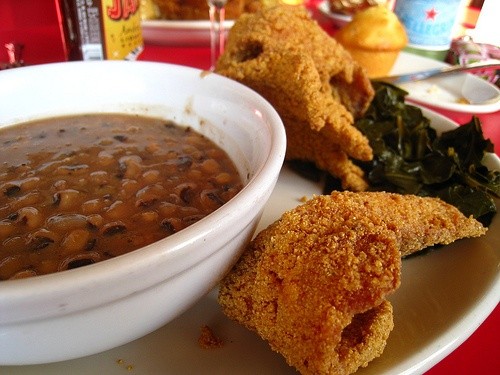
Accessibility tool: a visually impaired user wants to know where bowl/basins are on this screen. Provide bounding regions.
[0,59,287,364]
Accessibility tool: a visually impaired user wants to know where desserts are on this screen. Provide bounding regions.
[331,6,408,79]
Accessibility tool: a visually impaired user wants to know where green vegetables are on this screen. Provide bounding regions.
[289,80,500,229]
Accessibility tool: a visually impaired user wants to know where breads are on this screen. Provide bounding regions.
[140,0,245,21]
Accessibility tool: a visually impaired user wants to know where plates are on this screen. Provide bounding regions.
[316,1,352,27]
[139,19,234,44]
[385,50,500,113]
[0,100,500,375]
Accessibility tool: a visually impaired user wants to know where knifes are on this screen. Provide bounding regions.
[374,59,500,84]
